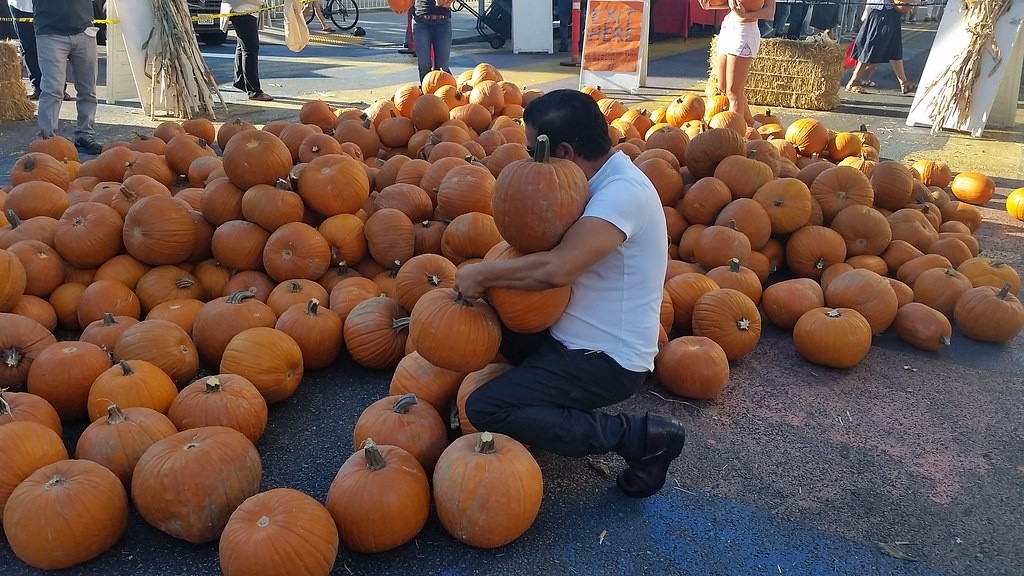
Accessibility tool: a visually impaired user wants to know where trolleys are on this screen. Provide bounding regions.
[449,0,507,49]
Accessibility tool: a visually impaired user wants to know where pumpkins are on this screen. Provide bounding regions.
[387,0,412,12]
[0,62,1024,576]
[736,0,765,11]
[893,0,915,13]
[706,0,727,6]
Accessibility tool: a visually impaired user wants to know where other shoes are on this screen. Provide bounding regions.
[753,120,762,129]
[844,80,867,94]
[249,90,274,101]
[901,84,917,96]
[860,79,876,87]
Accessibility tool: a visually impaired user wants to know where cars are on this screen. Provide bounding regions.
[187,0,234,45]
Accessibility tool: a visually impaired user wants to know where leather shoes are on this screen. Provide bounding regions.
[616,412,685,498]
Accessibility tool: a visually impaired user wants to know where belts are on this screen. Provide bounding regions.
[418,14,447,20]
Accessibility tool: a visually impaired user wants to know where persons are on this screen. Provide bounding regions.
[220,0,274,101]
[7,0,103,155]
[765,1,919,95]
[453,89,686,497]
[393,0,453,94]
[299,0,334,33]
[698,0,776,128]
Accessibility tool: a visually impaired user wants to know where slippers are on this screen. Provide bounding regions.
[322,27,336,32]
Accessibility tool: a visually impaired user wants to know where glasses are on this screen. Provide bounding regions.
[526,139,576,158]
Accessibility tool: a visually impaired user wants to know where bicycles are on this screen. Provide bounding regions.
[303,0,359,30]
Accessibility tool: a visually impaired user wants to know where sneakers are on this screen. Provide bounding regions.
[28,90,40,100]
[64,92,70,100]
[73,135,102,155]
